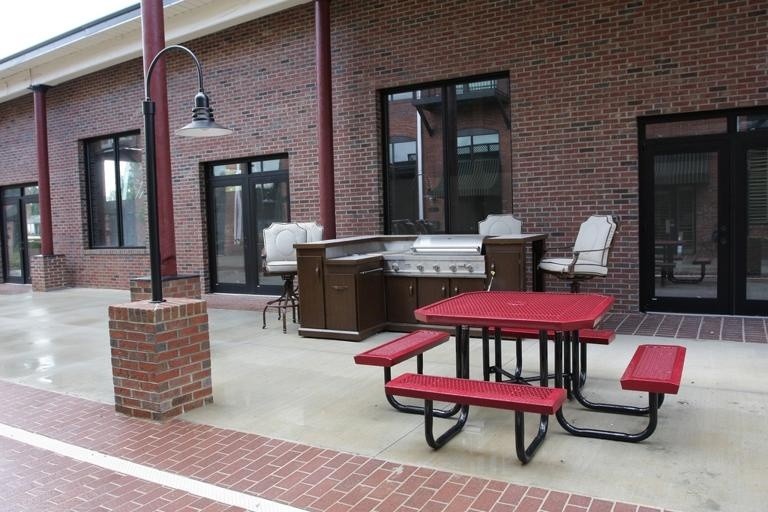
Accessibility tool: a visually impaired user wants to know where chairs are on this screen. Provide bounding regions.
[536,213,619,293]
[258,220,325,334]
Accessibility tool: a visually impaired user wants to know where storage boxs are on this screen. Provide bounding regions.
[654,255,712,287]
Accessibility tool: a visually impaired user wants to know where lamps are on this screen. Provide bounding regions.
[258,220,325,334]
[536,213,619,293]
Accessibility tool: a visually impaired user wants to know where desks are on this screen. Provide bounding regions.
[142,44,233,304]
[412,290,617,428]
[654,240,688,281]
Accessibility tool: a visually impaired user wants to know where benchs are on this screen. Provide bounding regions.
[354,326,687,464]
[654,255,712,287]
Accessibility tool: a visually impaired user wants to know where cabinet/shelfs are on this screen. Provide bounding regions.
[386,275,417,323]
[487,252,521,291]
[478,213,523,236]
[416,278,484,308]
[297,255,384,342]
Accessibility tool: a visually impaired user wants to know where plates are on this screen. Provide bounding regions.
[478,213,523,236]
[416,278,484,308]
[386,275,417,323]
[487,252,521,291]
[297,255,384,342]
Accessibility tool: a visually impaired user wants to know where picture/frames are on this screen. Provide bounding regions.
[412,290,617,428]
[654,240,688,281]
